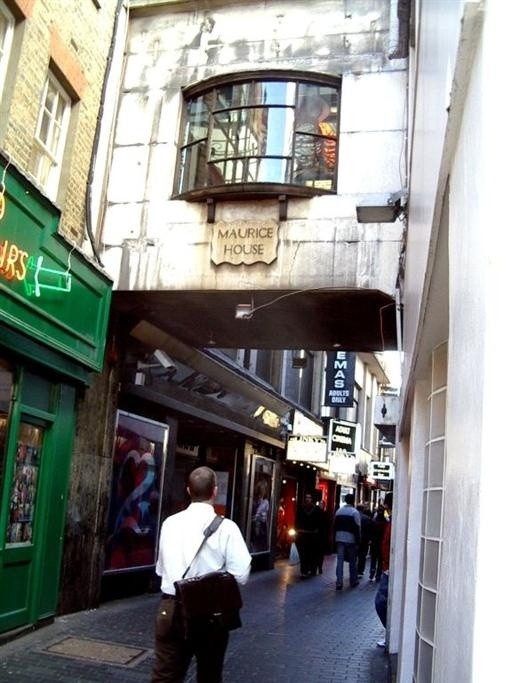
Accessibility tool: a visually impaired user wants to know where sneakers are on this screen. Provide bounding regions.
[376,638,385,647]
[335,574,375,589]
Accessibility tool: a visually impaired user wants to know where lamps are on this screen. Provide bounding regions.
[291,357,307,370]
[354,187,409,225]
[285,422,293,434]
[134,371,147,389]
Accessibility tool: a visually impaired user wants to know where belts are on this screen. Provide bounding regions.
[160,593,175,600]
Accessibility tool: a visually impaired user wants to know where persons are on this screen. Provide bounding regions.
[294,494,321,578]
[315,505,331,575]
[355,498,389,583]
[375,493,392,648]
[150,465,253,682]
[250,480,271,552]
[335,493,362,590]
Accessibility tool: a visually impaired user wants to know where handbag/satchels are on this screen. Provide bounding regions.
[288,541,299,565]
[171,570,242,640]
